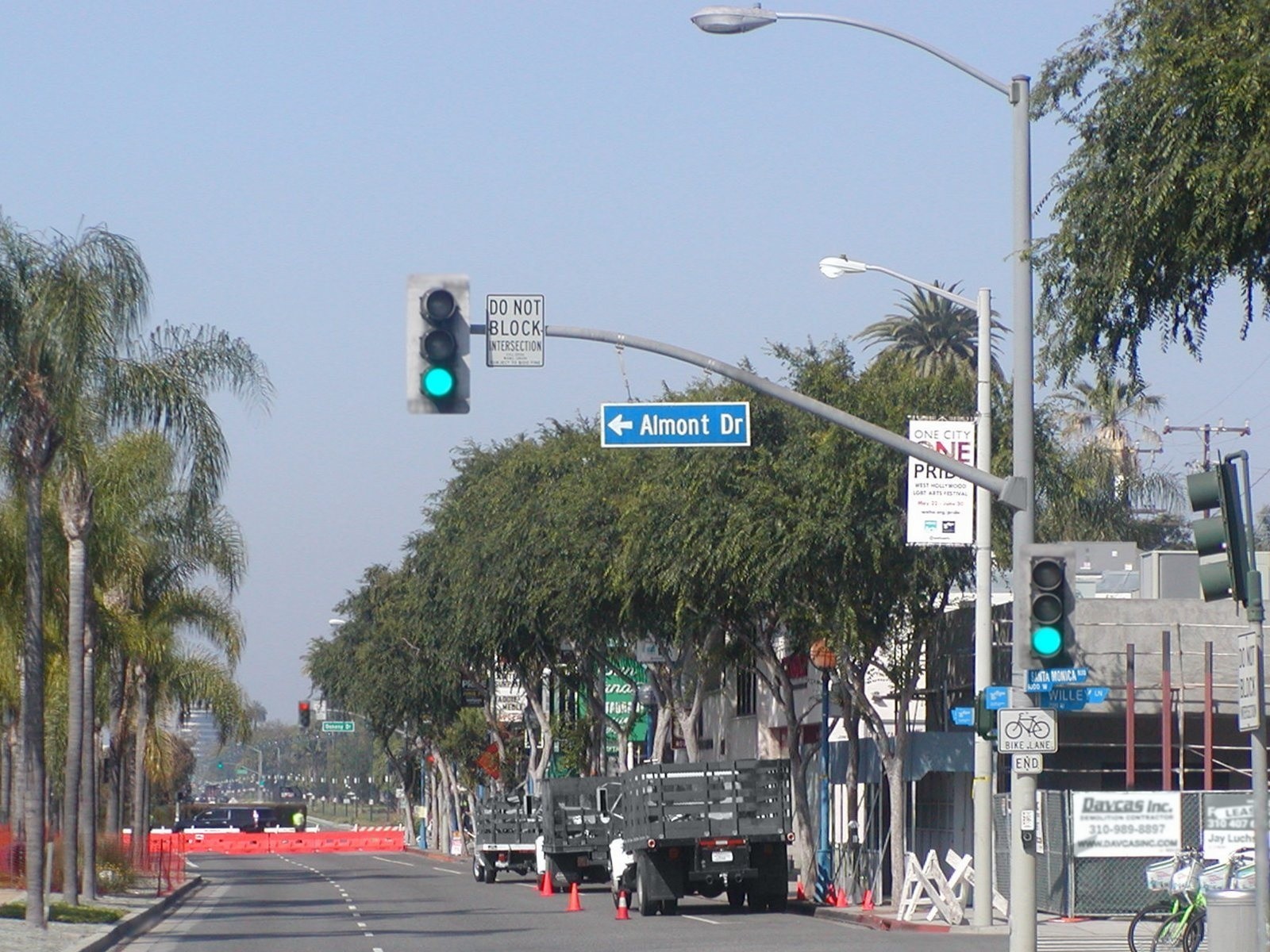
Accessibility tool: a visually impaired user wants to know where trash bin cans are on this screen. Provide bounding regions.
[1203,889,1260,951]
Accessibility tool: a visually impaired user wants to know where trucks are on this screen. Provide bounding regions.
[469,756,793,913]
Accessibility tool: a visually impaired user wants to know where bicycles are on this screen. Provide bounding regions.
[1127,842,1255,952]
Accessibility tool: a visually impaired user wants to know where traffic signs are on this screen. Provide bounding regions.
[598,404,752,448]
[1026,665,1110,712]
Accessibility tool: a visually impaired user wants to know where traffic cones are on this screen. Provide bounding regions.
[540,870,555,895]
[826,881,836,906]
[614,891,632,920]
[863,890,877,910]
[563,882,585,911]
[835,887,849,908]
[796,880,809,901]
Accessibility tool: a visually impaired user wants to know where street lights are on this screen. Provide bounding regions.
[816,258,995,935]
[812,635,838,912]
[684,5,1043,951]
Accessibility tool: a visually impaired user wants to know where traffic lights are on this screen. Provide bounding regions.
[1027,555,1070,662]
[218,761,223,769]
[409,275,475,418]
[1182,448,1244,607]
[298,699,310,727]
